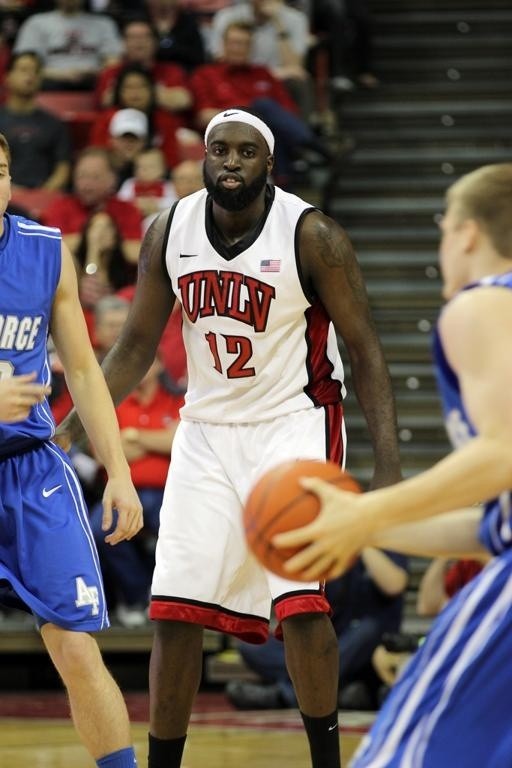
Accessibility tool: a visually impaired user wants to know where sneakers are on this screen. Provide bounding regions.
[226,681,279,709]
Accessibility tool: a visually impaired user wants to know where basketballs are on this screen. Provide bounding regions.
[243,459,361,582]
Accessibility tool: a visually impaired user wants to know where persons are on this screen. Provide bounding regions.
[0,133,146,765]
[0,2,340,628]
[223,539,410,712]
[413,551,492,620]
[36,103,410,765]
[266,159,510,767]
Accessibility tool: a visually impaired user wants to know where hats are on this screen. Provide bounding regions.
[108,110,149,139]
